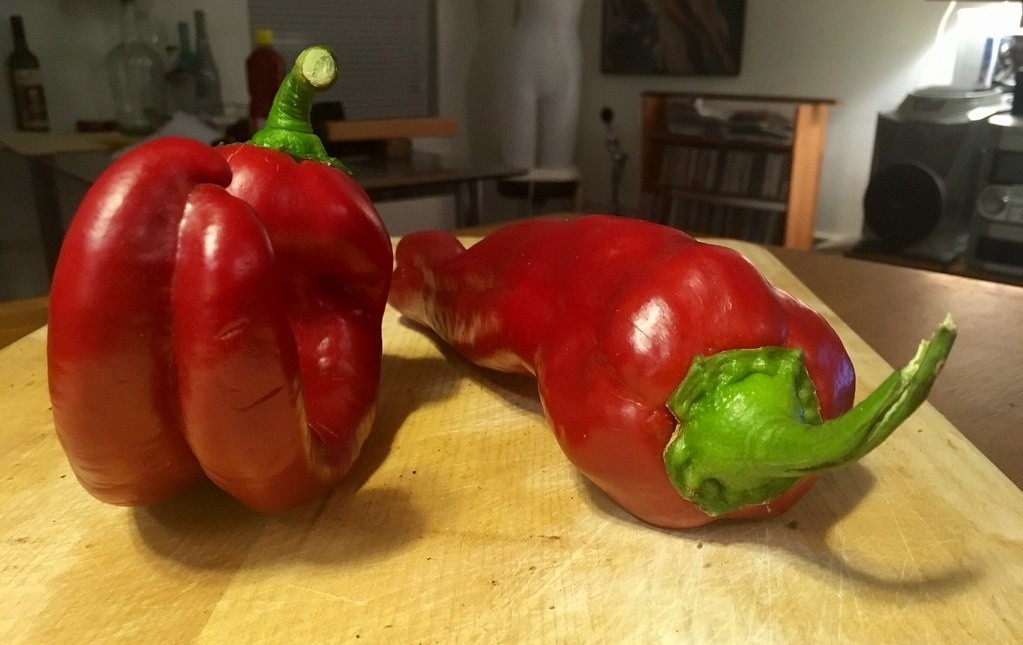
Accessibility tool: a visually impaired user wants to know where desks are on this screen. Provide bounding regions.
[44,154,524,237]
[1,247,1023,645]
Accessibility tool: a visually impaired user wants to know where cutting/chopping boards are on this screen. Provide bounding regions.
[0,238,1022,645]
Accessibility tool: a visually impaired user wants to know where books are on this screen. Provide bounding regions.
[653,103,793,246]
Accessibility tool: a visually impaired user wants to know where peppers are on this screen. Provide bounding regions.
[390,213,960,530]
[47,47,393,516]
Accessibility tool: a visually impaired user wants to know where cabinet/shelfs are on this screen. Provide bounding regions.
[639,91,835,251]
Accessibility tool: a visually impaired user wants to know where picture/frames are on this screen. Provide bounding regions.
[597,0,749,78]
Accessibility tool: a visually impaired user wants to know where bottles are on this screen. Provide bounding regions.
[169,21,196,114]
[193,9,225,118]
[7,14,51,131]
[244,29,286,129]
[104,0,169,135]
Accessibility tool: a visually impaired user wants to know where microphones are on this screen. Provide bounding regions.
[599,108,621,162]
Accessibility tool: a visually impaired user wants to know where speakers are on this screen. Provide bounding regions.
[860,82,1016,263]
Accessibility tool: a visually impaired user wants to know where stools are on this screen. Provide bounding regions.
[498,164,581,219]
